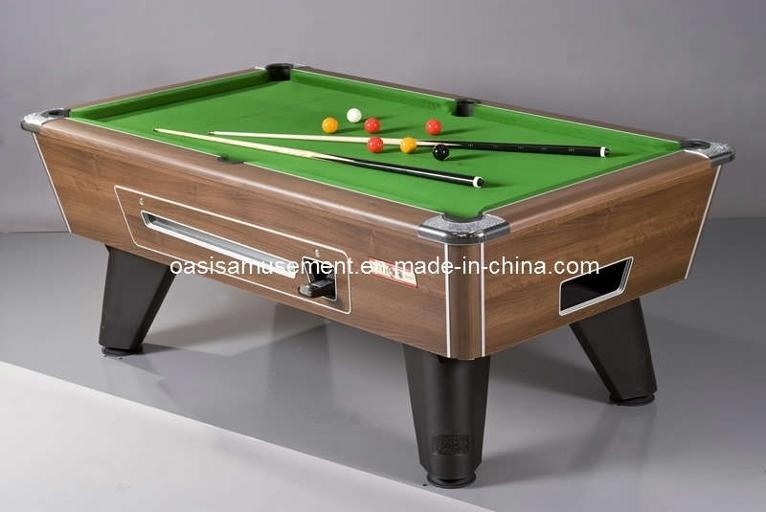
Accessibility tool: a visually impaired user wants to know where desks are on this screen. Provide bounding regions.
[20,62,735,490]
